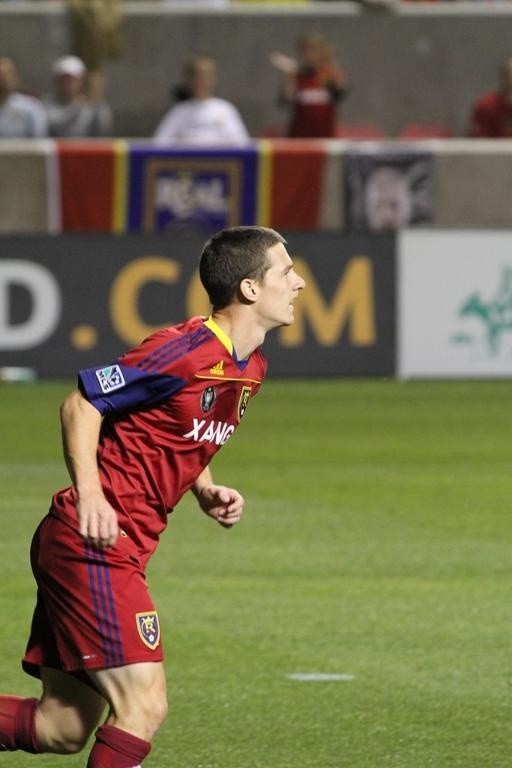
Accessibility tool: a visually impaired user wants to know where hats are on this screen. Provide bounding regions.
[53,54,87,81]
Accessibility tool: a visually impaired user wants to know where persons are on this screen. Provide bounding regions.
[153,55,250,140]
[0,225,307,768]
[469,54,512,140]
[1,53,113,139]
[270,31,351,139]
